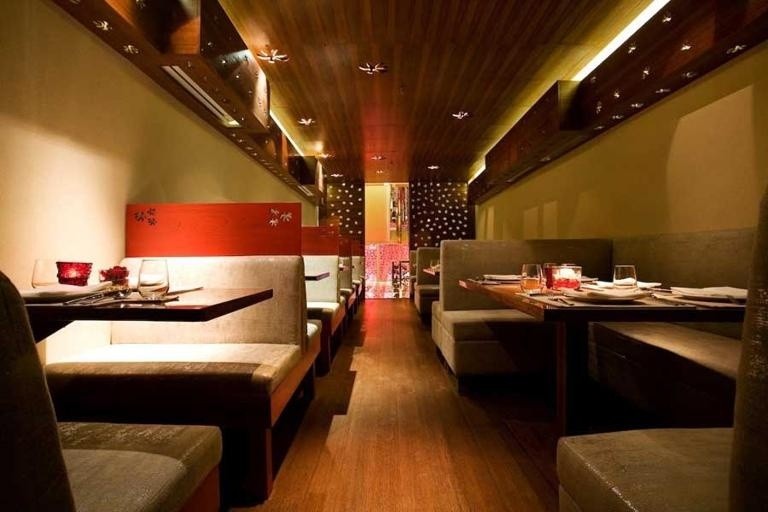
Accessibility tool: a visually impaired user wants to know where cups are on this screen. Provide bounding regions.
[613,264,637,286]
[542,262,556,289]
[551,265,582,290]
[519,263,545,294]
[137,259,170,299]
[31,259,55,288]
[57,260,93,286]
[430,259,440,268]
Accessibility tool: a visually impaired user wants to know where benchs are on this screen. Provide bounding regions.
[556,189,768,512]
[304,255,340,373]
[416,247,440,314]
[440,238,613,394]
[0,271,225,512]
[409,251,416,297]
[44,255,304,501]
[339,256,352,325]
[590,229,750,380]
[352,256,365,296]
